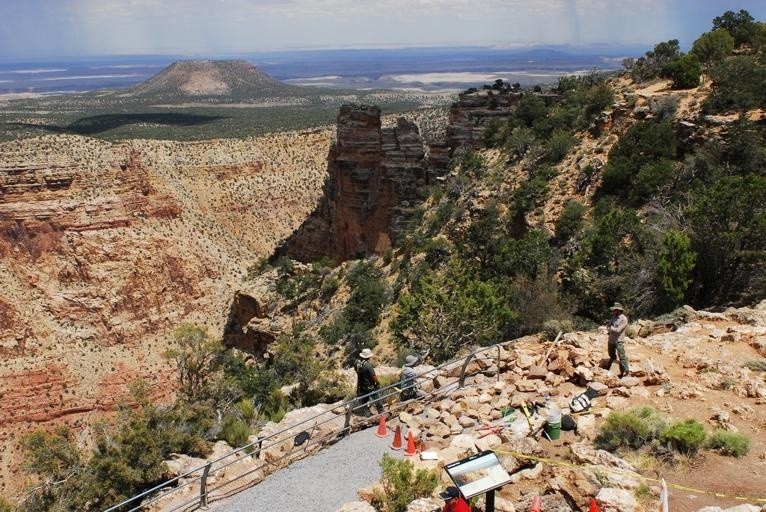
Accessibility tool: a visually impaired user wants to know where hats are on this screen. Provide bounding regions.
[359,348,373,359]
[610,302,624,311]
[405,355,418,366]
[439,486,459,499]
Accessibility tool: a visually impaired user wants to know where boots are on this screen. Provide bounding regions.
[621,370,629,377]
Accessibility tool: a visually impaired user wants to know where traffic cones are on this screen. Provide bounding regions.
[390,426,401,450]
[403,432,415,456]
[530,495,540,512]
[421,443,428,453]
[589,498,599,512]
[375,415,388,439]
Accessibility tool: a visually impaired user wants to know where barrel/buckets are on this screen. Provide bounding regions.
[548,414,561,440]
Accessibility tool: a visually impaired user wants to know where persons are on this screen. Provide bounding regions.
[354,348,385,428]
[598,301,630,376]
[397,355,435,398]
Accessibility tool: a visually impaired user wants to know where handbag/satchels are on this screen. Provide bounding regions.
[367,385,374,391]
